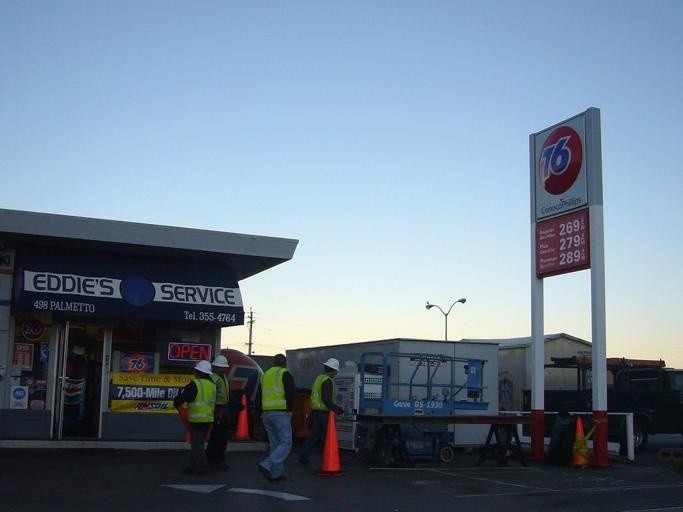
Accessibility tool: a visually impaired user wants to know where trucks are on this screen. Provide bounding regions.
[522,354,683,455]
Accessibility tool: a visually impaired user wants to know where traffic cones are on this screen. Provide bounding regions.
[234,393,251,441]
[318,410,346,479]
[574,417,587,469]
[176,400,192,444]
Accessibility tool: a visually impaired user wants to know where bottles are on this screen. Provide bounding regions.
[61,345,87,425]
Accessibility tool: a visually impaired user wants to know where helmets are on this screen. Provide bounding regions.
[194,360,213,374]
[323,358,339,371]
[212,355,229,367]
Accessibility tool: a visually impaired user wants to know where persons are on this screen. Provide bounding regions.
[247,400,256,440]
[307,357,345,465]
[173,360,216,478]
[255,355,296,483]
[205,355,245,471]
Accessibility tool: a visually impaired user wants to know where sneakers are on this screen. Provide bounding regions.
[257,464,287,480]
